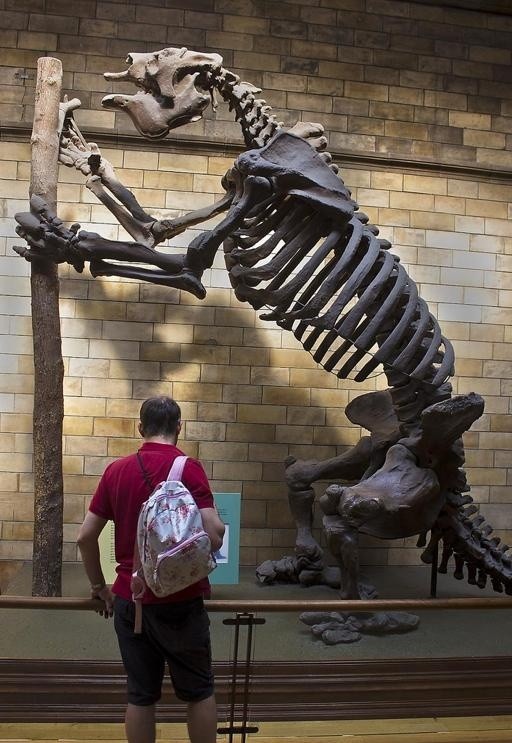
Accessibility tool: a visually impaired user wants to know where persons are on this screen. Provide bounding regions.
[78,396,225,743]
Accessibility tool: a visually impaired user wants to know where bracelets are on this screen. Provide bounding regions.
[90,580,106,590]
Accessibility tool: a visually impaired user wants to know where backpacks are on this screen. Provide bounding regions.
[137,481,217,598]
